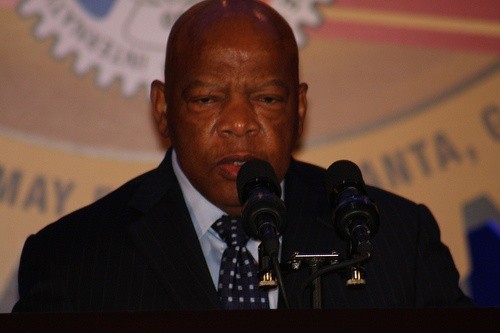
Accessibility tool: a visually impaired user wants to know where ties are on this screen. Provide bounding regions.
[211,214,269,310]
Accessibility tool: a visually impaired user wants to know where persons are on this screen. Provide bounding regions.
[12,0,476,310]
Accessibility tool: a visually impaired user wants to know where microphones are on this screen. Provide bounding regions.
[236,159,286,257]
[325,160,381,256]
